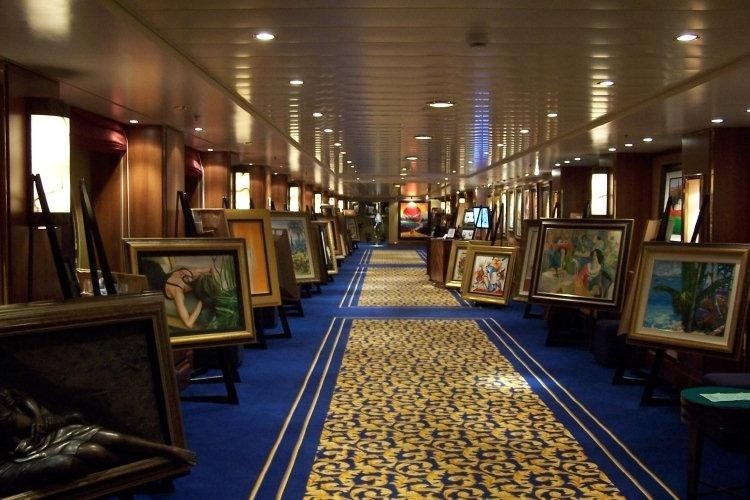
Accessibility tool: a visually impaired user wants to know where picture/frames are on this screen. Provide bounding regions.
[398,200,431,241]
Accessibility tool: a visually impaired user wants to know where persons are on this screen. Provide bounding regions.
[0,456,90,496]
[143,258,223,328]
[373,210,384,247]
[0,382,200,467]
[547,234,617,299]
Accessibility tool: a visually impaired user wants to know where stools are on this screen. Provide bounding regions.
[680,386,749,499]
